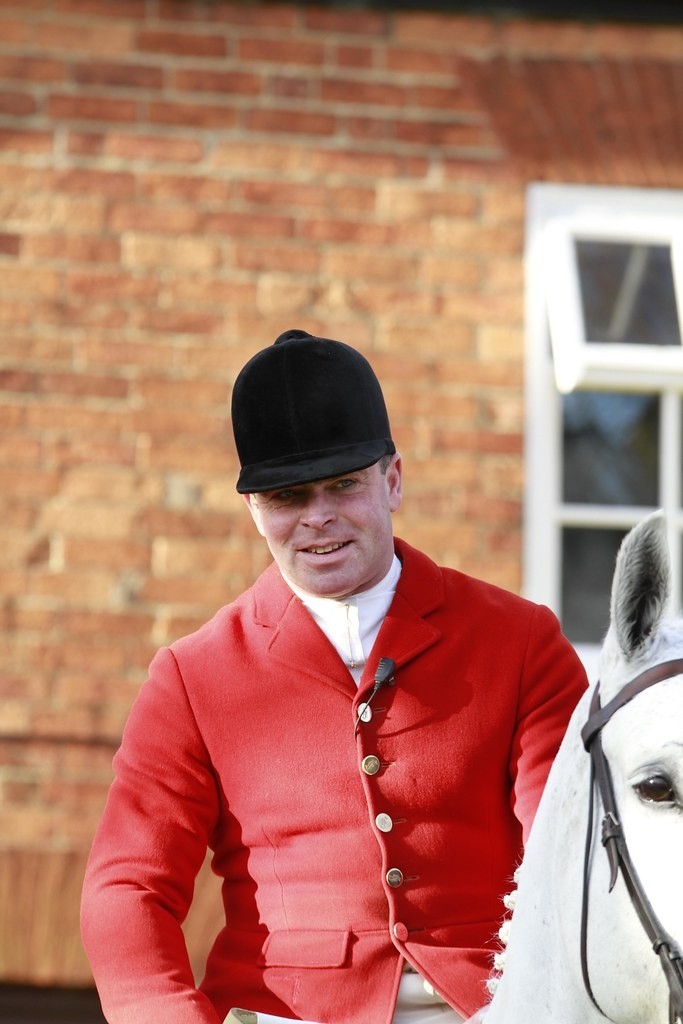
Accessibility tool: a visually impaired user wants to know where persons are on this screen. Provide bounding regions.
[80,329,588,1024]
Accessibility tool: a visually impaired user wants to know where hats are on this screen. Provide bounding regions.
[231,330,397,494]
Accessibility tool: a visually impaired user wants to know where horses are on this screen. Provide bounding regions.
[481,509,683,1023]
